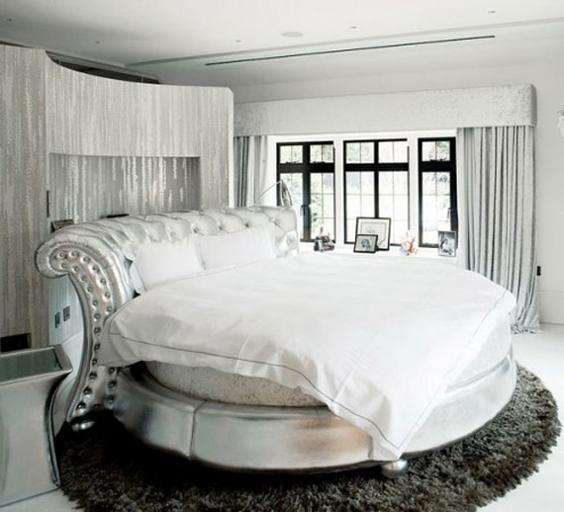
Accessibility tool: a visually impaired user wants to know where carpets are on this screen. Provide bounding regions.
[49,359,561,512]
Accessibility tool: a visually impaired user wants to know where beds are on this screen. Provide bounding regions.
[35,204,521,471]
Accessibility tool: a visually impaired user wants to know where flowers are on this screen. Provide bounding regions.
[400,231,420,255]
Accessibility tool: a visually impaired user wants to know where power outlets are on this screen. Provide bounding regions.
[50,311,64,329]
[63,306,75,323]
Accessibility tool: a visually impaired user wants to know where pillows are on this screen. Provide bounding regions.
[123,233,204,297]
[196,224,278,274]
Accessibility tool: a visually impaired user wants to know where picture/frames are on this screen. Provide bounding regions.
[355,217,391,251]
[438,231,457,257]
[353,234,379,254]
